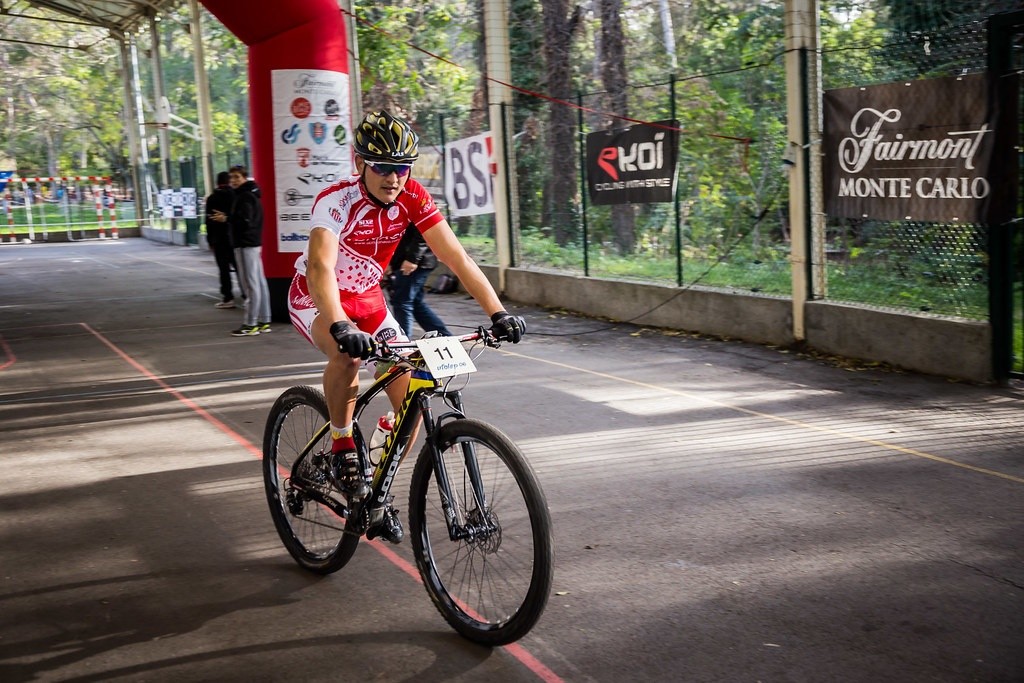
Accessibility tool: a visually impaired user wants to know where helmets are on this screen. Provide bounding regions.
[353,108,419,161]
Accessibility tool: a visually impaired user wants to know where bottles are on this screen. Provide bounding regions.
[369,411,396,465]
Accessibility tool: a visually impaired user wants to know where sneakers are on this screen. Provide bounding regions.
[214,300,234,308]
[379,503,404,544]
[229,324,260,336]
[257,322,272,332]
[330,449,369,498]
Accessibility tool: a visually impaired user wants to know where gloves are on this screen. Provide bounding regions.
[330,320,377,360]
[491,310,528,344]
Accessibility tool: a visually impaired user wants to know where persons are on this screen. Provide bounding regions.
[391,215,457,339]
[206,173,250,308]
[287,109,527,542]
[209,165,273,335]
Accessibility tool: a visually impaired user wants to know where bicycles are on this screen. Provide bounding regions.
[259,319,555,650]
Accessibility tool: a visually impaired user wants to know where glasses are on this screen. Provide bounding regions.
[363,161,414,178]
